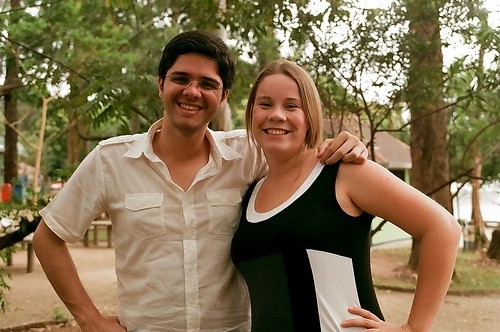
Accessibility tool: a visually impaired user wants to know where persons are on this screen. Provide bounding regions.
[230,58,462,332]
[32,30,368,332]
[0,177,14,203]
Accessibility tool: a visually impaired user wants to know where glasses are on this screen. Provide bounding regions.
[159,73,225,91]
